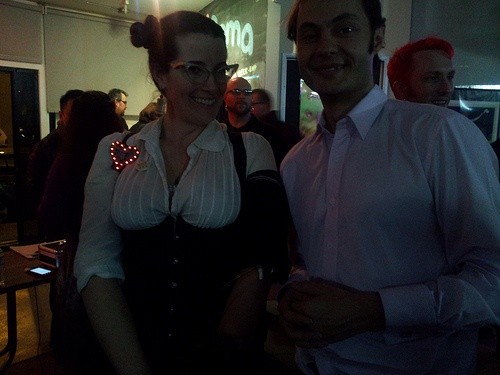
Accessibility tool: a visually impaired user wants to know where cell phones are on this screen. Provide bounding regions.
[26,266,52,276]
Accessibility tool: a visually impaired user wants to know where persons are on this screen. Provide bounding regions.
[71,11,305,375]
[224,77,303,166]
[387,37,455,108]
[267,0,500,375]
[16,89,165,270]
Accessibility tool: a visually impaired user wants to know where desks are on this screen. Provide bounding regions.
[0,239,67,375]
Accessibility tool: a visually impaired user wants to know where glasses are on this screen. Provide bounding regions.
[225,89,253,96]
[121,100,127,104]
[168,62,239,85]
